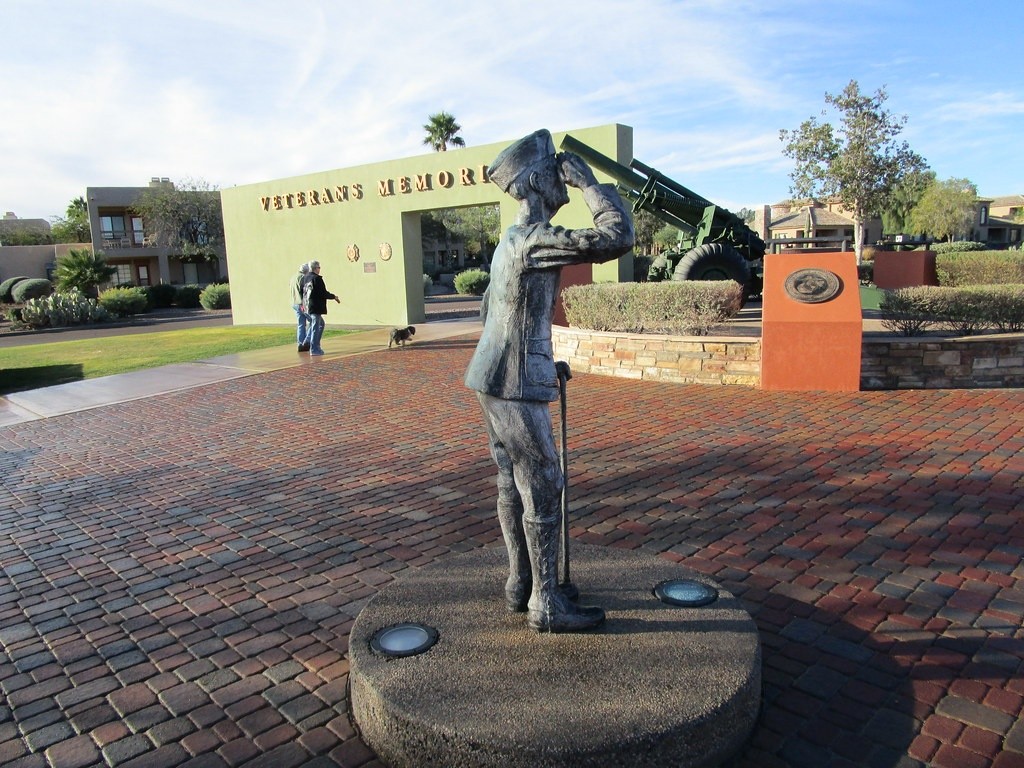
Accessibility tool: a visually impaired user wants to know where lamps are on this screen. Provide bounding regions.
[470,254,476,260]
[452,251,457,258]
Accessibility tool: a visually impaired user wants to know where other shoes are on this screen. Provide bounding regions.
[302,340,310,348]
[298,345,305,351]
[310,349,324,356]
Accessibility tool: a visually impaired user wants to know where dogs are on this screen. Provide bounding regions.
[388,325,415,348]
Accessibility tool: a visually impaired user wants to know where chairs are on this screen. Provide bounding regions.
[100,233,158,249]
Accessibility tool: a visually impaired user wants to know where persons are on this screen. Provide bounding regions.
[290,261,340,356]
[460,130,636,629]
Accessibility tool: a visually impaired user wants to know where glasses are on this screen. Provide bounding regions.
[315,267,320,268]
